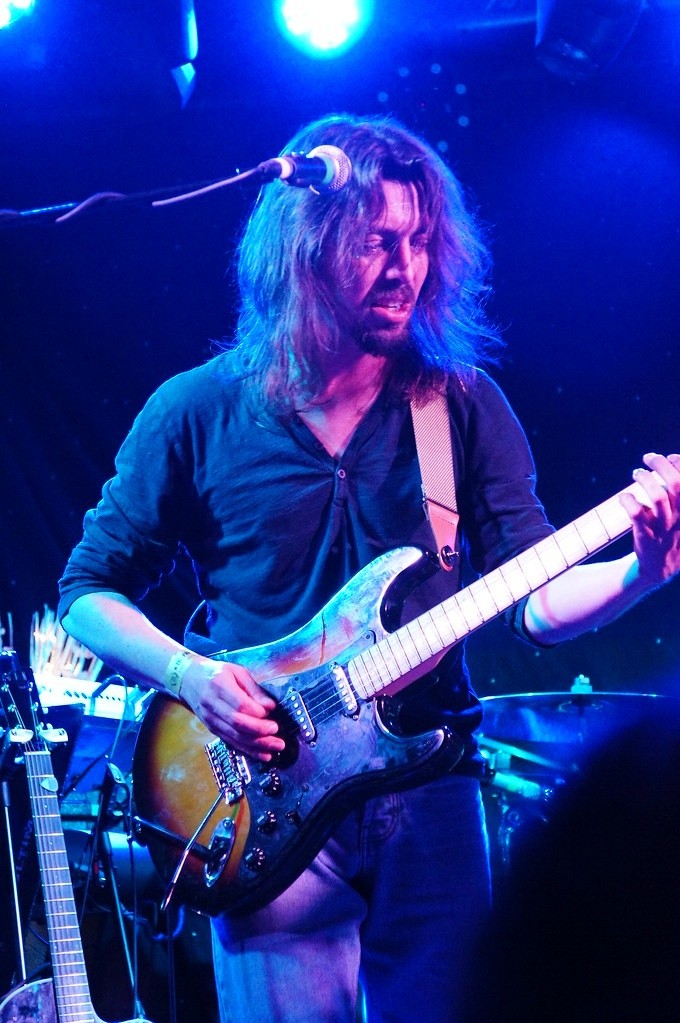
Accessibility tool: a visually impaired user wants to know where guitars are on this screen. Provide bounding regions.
[0,667,154,1023]
[130,469,680,922]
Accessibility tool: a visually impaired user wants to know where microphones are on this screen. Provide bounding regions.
[259,144,352,195]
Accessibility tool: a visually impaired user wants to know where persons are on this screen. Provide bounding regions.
[47,113,679,1023]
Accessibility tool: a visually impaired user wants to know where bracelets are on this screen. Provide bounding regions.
[163,649,198,699]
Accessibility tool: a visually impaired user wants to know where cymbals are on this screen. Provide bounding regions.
[478,691,672,743]
